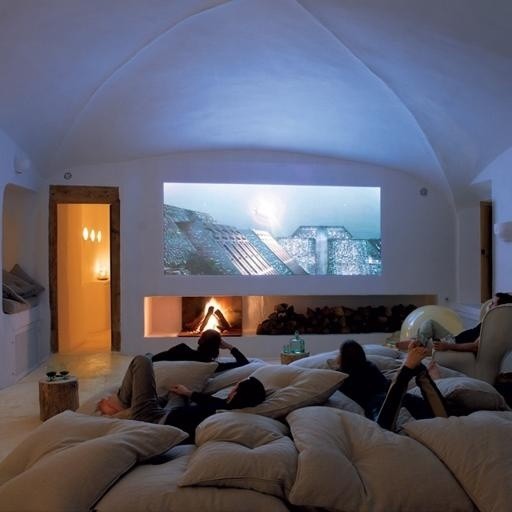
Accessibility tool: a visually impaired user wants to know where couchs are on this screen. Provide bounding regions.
[431,298,512,386]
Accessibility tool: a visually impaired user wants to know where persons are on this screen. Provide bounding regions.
[331,341,451,433]
[145,329,250,374]
[396,292,512,352]
[96,354,267,446]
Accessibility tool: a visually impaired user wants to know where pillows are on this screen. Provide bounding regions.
[212,363,350,417]
[402,410,512,512]
[0,409,190,512]
[177,411,299,499]
[408,378,507,409]
[202,356,270,395]
[76,360,220,424]
[287,344,401,370]
[11,264,45,298]
[4,269,36,298]
[285,406,475,511]
[93,442,288,512]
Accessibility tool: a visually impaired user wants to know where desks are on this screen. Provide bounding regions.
[39,376,79,422]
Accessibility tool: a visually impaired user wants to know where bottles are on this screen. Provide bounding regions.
[290,329,305,354]
